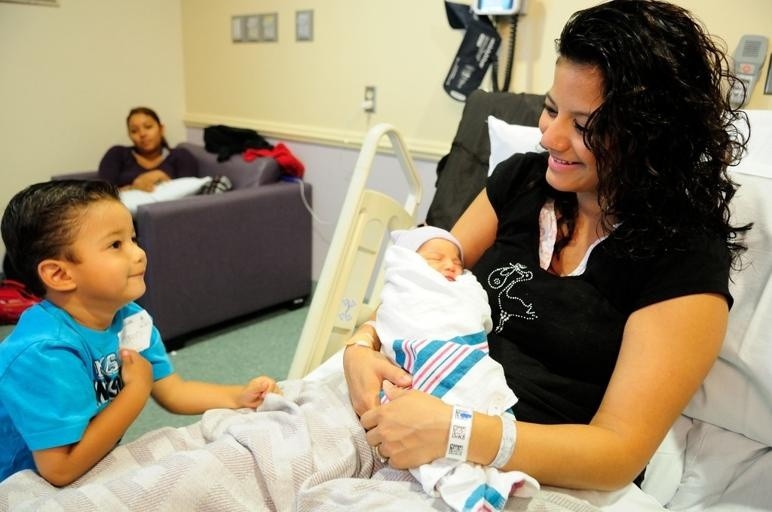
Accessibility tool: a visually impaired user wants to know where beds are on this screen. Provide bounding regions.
[0,89,770,512]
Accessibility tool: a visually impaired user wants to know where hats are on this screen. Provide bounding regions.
[391,224,466,266]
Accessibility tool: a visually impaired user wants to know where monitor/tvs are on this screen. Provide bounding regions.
[472,1,521,18]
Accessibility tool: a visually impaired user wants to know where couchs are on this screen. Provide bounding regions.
[48,144,315,355]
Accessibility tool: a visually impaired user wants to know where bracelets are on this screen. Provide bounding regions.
[445,405,473,463]
[489,412,517,469]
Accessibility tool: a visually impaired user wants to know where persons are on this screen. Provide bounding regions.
[344,0,753,492]
[98,107,199,191]
[388,224,525,496]
[0,178,284,487]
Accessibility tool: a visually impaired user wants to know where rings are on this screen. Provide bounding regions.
[374,447,381,462]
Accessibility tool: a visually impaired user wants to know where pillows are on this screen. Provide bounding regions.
[483,110,769,447]
[113,177,212,218]
